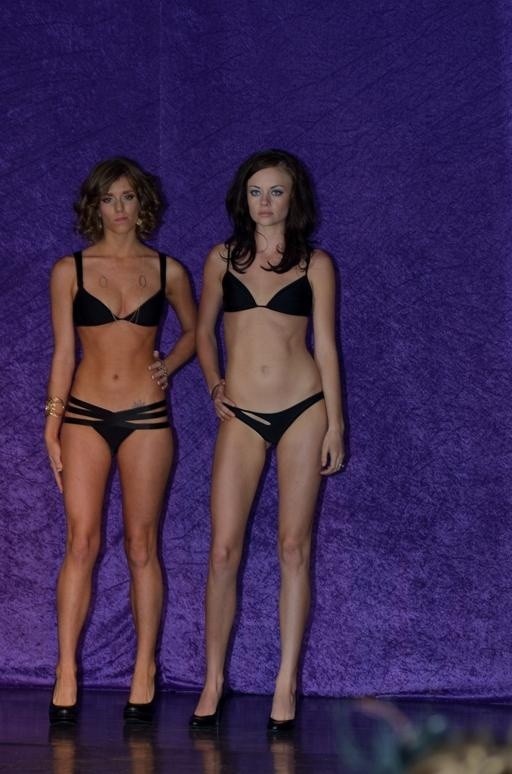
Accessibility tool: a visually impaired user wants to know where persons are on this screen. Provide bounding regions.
[45,153,199,730]
[188,147,347,737]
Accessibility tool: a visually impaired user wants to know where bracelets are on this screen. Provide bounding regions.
[44,396,65,418]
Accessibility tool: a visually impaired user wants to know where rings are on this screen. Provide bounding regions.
[339,462,345,470]
[162,368,167,375]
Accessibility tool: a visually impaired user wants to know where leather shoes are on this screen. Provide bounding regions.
[48,670,81,726]
[267,682,297,733]
[189,680,225,728]
[122,671,157,724]
